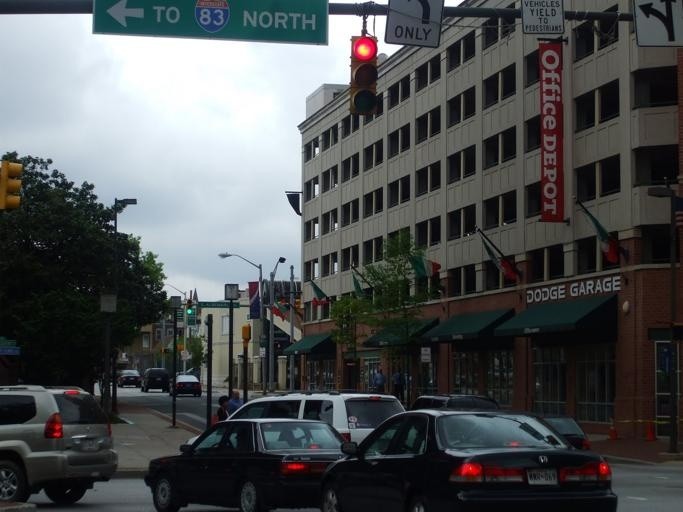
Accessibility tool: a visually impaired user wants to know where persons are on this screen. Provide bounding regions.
[216,395,229,421]
[227,388,242,415]
[392,366,404,401]
[374,369,386,394]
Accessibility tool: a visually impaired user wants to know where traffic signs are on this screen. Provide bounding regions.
[90,0,330,46]
[519,0,567,34]
[1,346,21,356]
[382,0,444,48]
[0,337,17,346]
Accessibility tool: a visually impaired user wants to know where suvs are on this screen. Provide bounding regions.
[0,384,117,505]
[141,368,169,392]
[411,393,499,414]
[183,390,408,448]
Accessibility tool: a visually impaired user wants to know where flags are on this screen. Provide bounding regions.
[310,281,331,304]
[481,239,518,281]
[584,211,619,264]
[351,272,367,297]
[271,296,289,321]
[407,255,441,278]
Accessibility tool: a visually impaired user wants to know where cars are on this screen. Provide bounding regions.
[144,416,348,512]
[537,413,590,451]
[169,375,201,398]
[116,370,141,389]
[320,408,617,511]
[175,368,200,379]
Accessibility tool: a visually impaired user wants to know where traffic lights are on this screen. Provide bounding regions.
[0,157,25,214]
[187,299,194,316]
[240,324,251,341]
[348,36,378,118]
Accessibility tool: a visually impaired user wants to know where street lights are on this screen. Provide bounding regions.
[267,257,292,391]
[645,184,680,454]
[218,252,267,397]
[160,283,186,376]
